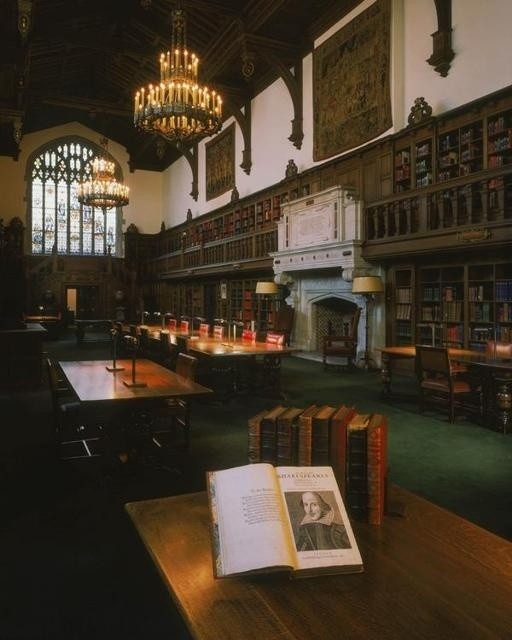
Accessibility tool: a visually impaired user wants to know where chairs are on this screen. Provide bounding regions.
[47,357,73,413]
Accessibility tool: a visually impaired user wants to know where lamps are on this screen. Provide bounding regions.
[75,0,224,213]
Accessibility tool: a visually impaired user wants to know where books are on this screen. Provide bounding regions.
[391,110,512,351]
[205,466,365,582]
[247,401,388,527]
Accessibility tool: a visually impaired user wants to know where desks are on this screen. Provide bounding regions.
[109,323,297,402]
